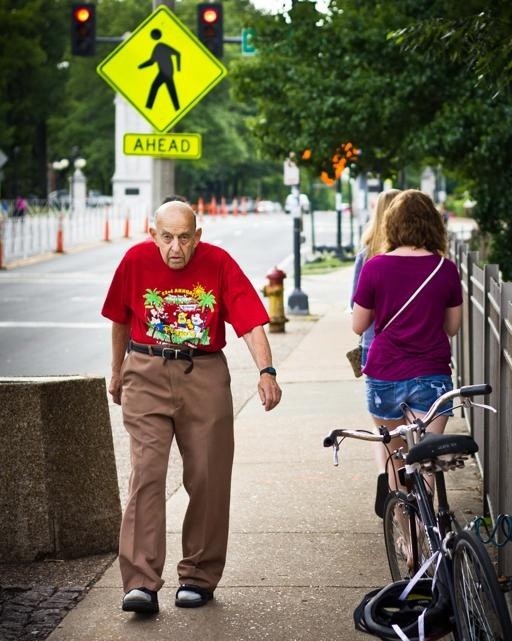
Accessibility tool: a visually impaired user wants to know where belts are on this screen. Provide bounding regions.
[128,340,217,374]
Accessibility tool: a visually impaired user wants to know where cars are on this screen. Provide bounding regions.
[45,185,114,208]
[284,194,312,214]
[216,197,280,215]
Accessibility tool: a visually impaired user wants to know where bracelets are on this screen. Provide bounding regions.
[259,367,277,378]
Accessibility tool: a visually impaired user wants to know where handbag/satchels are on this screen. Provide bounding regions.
[345,345,363,378]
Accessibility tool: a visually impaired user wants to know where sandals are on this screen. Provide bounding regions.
[122,587,159,614]
[175,583,213,608]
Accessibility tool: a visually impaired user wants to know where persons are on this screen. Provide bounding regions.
[350,188,466,573]
[13,192,26,217]
[99,198,284,617]
[347,186,405,367]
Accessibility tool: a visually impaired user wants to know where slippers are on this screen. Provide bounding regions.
[395,536,414,578]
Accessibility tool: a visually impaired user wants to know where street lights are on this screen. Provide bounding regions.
[50,154,91,220]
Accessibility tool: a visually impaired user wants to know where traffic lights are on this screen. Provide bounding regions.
[68,0,95,56]
[194,0,226,58]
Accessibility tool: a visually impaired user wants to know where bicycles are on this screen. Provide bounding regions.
[319,383,512,641]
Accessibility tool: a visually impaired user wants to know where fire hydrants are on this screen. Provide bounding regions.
[260,263,290,333]
